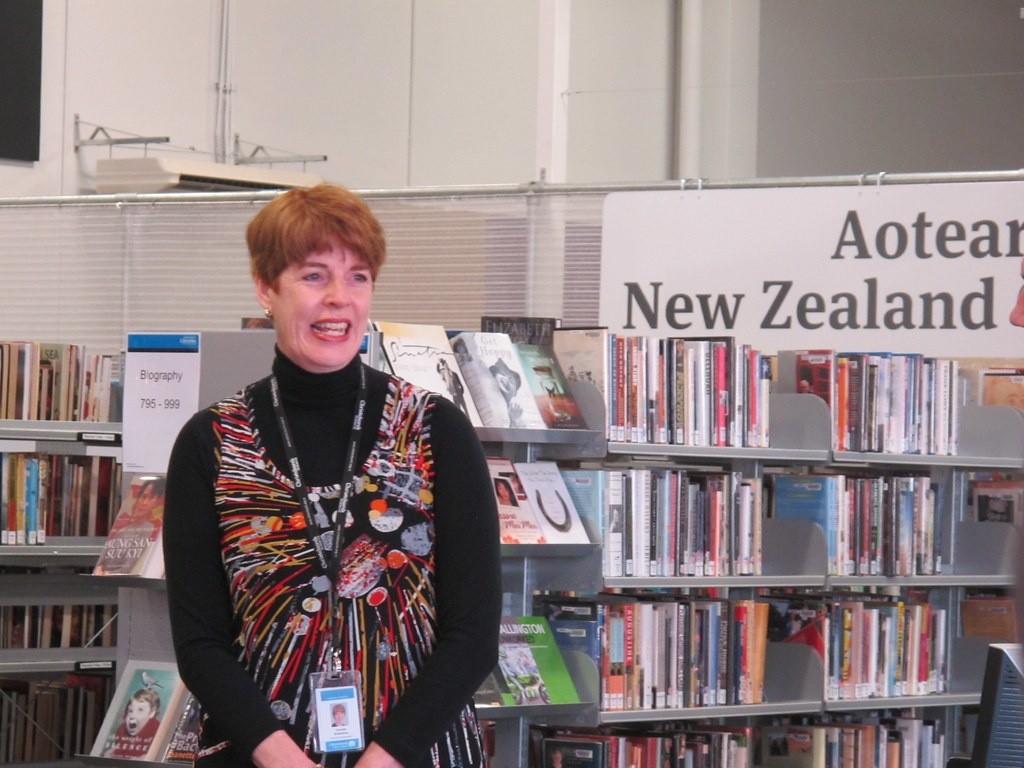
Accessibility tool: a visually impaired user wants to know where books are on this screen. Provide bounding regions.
[354,316,588,427]
[962,367,1024,643]
[756,590,947,768]
[770,349,959,579]
[0,340,112,422]
[88,659,203,767]
[89,475,168,578]
[553,329,771,449]
[528,717,750,768]
[470,616,580,708]
[601,466,765,578]
[530,588,771,711]
[0,451,122,546]
[486,455,607,544]
[0,675,114,763]
[0,605,118,649]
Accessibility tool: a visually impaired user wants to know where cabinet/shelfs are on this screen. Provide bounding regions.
[0,336,124,768]
[75,336,612,768]
[535,338,1024,768]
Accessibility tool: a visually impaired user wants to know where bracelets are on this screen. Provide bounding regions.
[314,763,322,768]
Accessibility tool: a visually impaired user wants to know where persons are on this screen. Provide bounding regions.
[451,338,473,367]
[330,704,346,727]
[162,182,503,768]
[784,604,805,636]
[798,379,811,393]
[490,357,528,427]
[104,688,160,761]
[93,477,166,573]
[494,478,519,507]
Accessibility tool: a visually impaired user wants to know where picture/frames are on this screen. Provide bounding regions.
[89,658,191,762]
